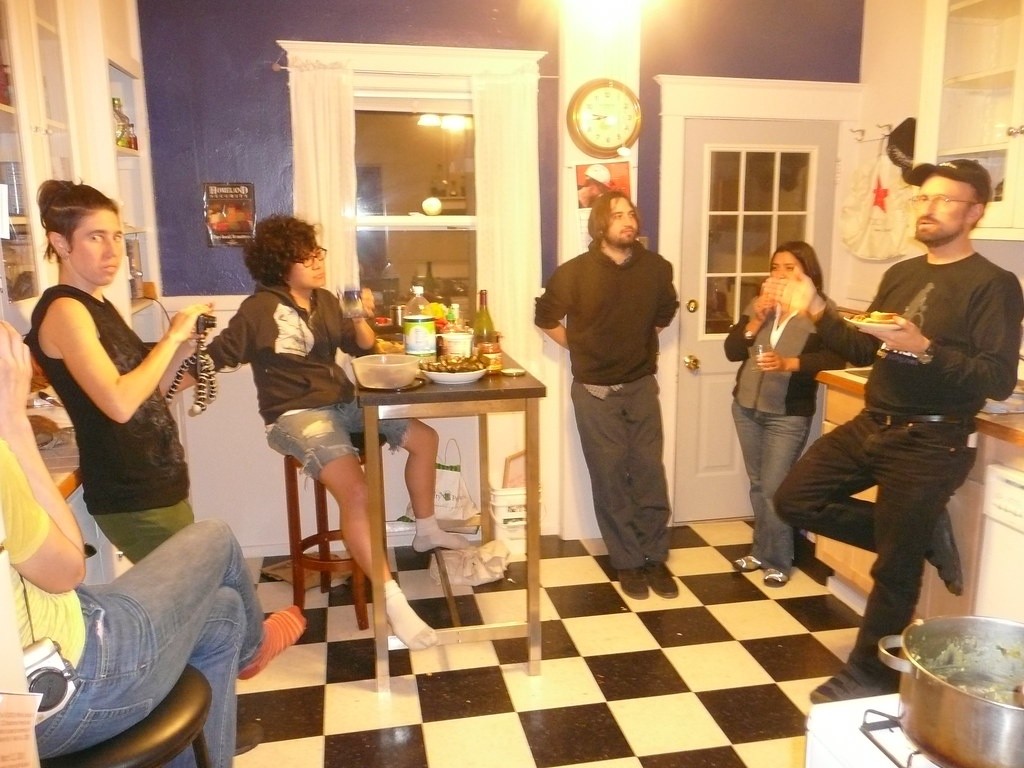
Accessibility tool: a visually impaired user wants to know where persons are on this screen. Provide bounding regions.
[534,191,680,600]
[762,159,1024,704]
[724,240,846,585]
[578,164,611,209]
[25,180,212,565]
[173,214,469,651]
[0,321,307,768]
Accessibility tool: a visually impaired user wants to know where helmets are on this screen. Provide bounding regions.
[585,164,611,190]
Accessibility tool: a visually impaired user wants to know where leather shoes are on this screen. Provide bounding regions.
[644,561,678,599]
[618,567,649,600]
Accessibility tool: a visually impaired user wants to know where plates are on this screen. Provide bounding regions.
[421,363,486,384]
[842,315,901,331]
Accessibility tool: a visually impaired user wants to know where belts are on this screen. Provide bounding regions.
[867,410,963,424]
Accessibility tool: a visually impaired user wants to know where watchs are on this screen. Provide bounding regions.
[918,339,938,365]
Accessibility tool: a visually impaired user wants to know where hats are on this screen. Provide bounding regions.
[909,159,990,200]
[886,117,916,181]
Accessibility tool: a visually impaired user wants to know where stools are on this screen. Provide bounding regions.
[42,660,214,768]
[283,430,385,632]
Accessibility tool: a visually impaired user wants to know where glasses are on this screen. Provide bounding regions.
[912,195,975,210]
[291,246,327,267]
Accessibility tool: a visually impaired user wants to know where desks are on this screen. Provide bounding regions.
[805,692,940,767]
[346,348,548,691]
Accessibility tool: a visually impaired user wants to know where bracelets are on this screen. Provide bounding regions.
[743,328,754,340]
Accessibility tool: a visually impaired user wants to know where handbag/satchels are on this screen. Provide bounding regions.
[405,436,478,521]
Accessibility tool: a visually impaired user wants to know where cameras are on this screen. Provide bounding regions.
[193,314,217,333]
[21,637,77,727]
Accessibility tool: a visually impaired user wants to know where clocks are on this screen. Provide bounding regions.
[567,81,641,156]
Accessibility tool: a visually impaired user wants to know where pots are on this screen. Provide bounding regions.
[876,614,1023,768]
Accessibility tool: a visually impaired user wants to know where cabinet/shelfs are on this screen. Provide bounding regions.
[116,142,156,315]
[904,0,1024,241]
[811,369,1024,618]
[64,482,132,587]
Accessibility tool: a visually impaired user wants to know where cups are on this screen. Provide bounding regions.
[748,345,771,372]
[389,305,406,326]
[342,291,369,318]
[443,333,472,357]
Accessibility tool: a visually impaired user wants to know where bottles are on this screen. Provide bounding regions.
[470,289,496,358]
[383,263,401,311]
[430,163,466,197]
[403,285,436,365]
[478,342,503,375]
[128,124,138,150]
[443,313,459,357]
[113,98,129,146]
[424,262,437,304]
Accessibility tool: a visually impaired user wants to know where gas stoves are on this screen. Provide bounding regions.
[807,692,940,768]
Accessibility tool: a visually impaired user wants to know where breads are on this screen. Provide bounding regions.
[862,311,897,325]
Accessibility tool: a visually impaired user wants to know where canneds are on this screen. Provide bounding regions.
[477,341,503,375]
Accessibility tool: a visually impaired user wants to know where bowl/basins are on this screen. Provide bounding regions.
[351,353,421,388]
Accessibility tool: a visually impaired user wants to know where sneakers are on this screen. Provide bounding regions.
[764,568,790,587]
[925,507,964,597]
[733,556,765,572]
[810,655,892,703]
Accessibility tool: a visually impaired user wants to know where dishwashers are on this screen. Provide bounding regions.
[972,464,1024,623]
